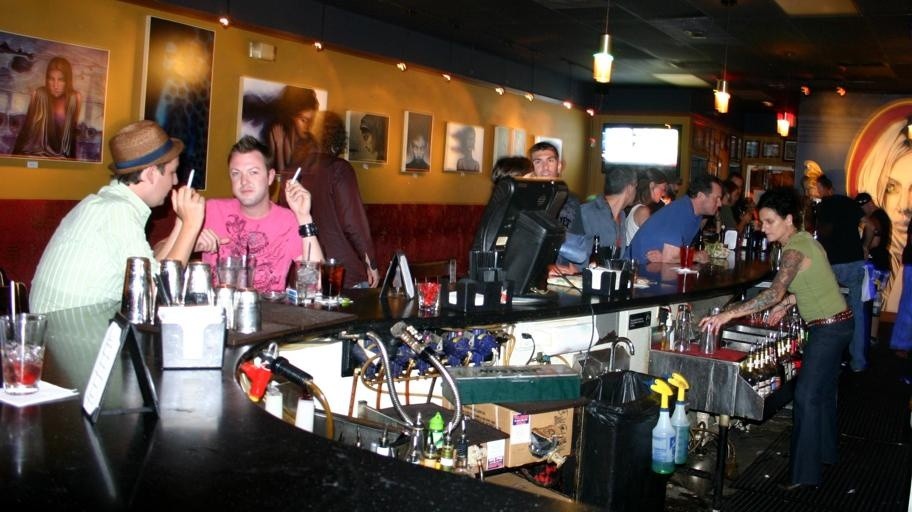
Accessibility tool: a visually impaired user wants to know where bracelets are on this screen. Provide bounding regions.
[298,223,319,236]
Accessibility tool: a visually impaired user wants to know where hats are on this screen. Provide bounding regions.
[108,120,183,175]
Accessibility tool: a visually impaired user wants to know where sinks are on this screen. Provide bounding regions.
[700,323,790,354]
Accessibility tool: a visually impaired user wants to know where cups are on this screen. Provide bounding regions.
[699,323,716,354]
[122,257,262,335]
[418,283,441,312]
[677,272,696,292]
[1,402,48,485]
[680,244,694,269]
[319,257,344,299]
[0,312,47,394]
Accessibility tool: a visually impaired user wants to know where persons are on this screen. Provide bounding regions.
[29,120,206,314]
[854,116,912,314]
[490,141,912,373]
[697,186,855,494]
[455,127,479,171]
[38,301,126,411]
[243,86,318,170]
[279,111,380,290]
[153,135,326,295]
[12,57,80,158]
[360,114,387,160]
[406,130,429,170]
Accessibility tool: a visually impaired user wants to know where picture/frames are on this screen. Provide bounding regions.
[688,121,796,203]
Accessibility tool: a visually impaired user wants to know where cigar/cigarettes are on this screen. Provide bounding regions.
[186,169,195,188]
[290,166,302,185]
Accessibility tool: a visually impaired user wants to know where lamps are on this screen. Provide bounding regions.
[712,1,731,116]
[591,1,615,84]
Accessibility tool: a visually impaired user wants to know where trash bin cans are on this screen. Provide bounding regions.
[578,370,678,512]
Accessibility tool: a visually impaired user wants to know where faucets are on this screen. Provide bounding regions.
[608,337,635,371]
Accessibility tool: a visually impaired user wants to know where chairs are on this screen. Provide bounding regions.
[0,269,30,315]
[405,257,459,288]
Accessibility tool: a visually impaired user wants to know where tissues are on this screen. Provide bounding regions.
[586,265,622,290]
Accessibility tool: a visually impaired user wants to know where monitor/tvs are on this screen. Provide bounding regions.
[601,122,682,183]
[468,175,568,305]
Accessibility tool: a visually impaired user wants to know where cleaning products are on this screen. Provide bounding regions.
[668,374,688,463]
[646,378,676,474]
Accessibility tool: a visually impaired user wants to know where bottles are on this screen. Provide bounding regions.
[708,306,725,349]
[336,411,475,481]
[741,306,809,398]
[618,248,634,298]
[589,235,603,268]
[661,304,691,352]
[696,232,705,252]
[296,260,319,296]
[738,225,771,253]
